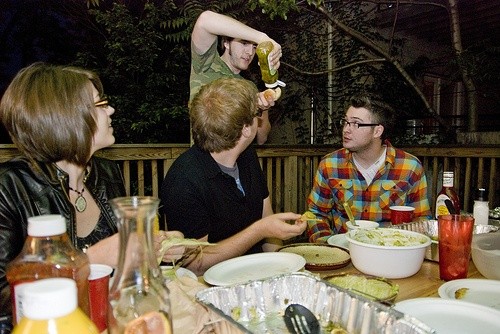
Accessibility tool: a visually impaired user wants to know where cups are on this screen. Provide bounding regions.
[87,263,114,334]
[389,205,416,225]
[438,213,475,282]
[435,172,461,220]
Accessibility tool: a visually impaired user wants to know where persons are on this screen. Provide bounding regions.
[187,10,282,146]
[0,60,186,334]
[305,94,432,245]
[158,77,307,276]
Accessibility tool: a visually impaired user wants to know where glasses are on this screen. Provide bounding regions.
[339,117,379,128]
[88,94,109,111]
[254,106,263,116]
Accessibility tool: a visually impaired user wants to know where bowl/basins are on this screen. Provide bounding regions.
[471,233,500,280]
[345,219,380,232]
[345,228,432,279]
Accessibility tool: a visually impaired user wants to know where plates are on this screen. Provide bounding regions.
[438,278,500,311]
[390,297,500,334]
[202,251,307,289]
[324,273,398,307]
[326,234,350,250]
[274,243,351,271]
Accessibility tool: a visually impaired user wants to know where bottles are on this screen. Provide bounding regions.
[8,276,100,334]
[6,214,92,325]
[106,193,174,334]
[472,188,489,233]
[257,41,286,90]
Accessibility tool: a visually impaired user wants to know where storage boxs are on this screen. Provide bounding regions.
[194,270,437,334]
[388,219,500,263]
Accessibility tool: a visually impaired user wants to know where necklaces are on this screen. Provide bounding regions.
[68,184,86,213]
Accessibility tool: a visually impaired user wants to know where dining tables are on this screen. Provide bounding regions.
[197,259,485,307]
